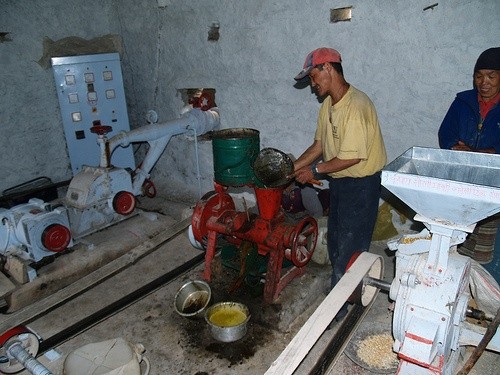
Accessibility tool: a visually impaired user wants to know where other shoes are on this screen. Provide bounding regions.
[327,307,352,328]
[322,283,334,294]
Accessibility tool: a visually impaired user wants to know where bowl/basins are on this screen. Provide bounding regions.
[205,300,252,342]
[174,279,214,319]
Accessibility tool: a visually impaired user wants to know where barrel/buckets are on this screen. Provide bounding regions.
[212,125,262,188]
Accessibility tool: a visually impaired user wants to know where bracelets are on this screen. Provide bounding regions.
[312,163,318,175]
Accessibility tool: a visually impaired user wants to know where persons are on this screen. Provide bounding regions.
[286,48,387,331]
[438,47,500,155]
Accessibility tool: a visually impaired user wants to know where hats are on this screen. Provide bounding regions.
[473,47,500,73]
[294,47,341,83]
